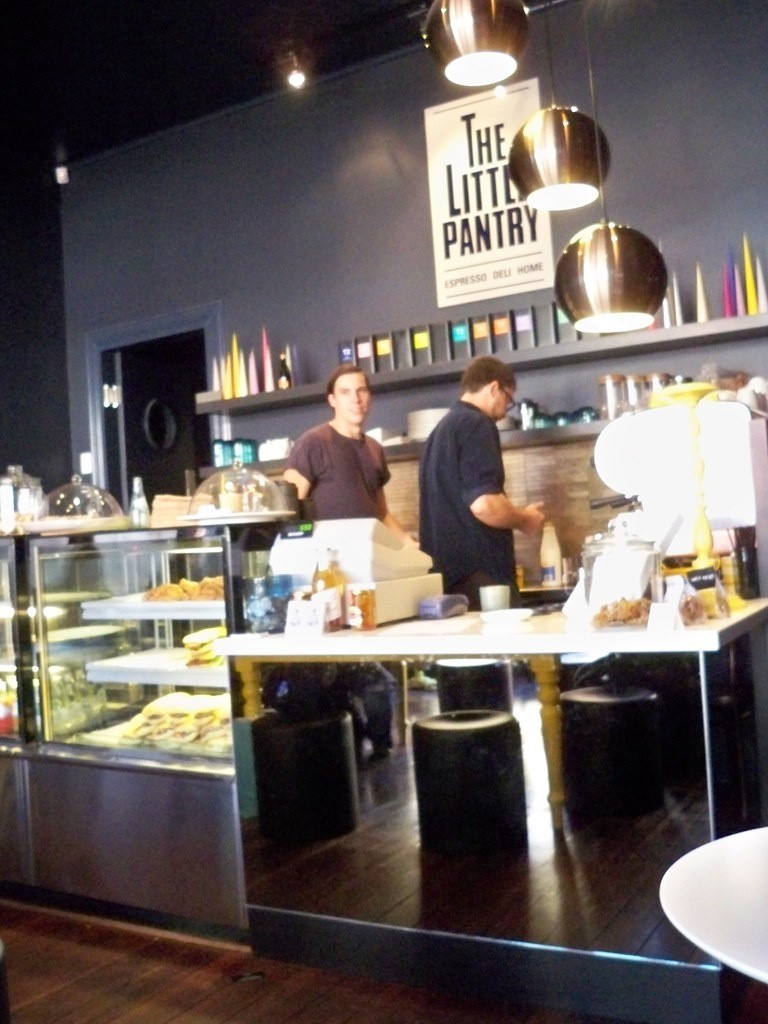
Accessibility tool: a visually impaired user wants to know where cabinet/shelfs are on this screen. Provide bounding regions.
[0,506,302,944]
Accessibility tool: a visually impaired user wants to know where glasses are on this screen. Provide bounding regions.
[500,385,516,411]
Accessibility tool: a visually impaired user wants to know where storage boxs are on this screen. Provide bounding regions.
[337,298,601,374]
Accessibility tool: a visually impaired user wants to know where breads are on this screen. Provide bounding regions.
[143,575,225,602]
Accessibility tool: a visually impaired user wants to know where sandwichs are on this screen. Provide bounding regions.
[121,691,236,756]
[181,626,227,666]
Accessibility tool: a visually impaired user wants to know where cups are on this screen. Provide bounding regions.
[242,575,294,635]
[479,584,510,611]
[350,582,376,632]
[562,557,582,597]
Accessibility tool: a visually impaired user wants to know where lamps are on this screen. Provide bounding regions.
[508,0,611,211]
[555,0,668,334]
[429,0,530,87]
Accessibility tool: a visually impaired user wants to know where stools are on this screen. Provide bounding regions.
[250,712,362,840]
[560,684,663,827]
[437,660,513,721]
[407,709,532,858]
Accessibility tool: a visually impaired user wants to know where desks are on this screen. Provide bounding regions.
[657,822,768,987]
[213,582,768,1024]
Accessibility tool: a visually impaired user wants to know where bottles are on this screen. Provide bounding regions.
[277,353,292,390]
[314,547,336,630]
[0,464,45,522]
[541,522,561,588]
[327,549,346,629]
[129,476,149,527]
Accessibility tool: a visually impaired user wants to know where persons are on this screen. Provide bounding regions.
[419,356,545,612]
[280,364,419,542]
